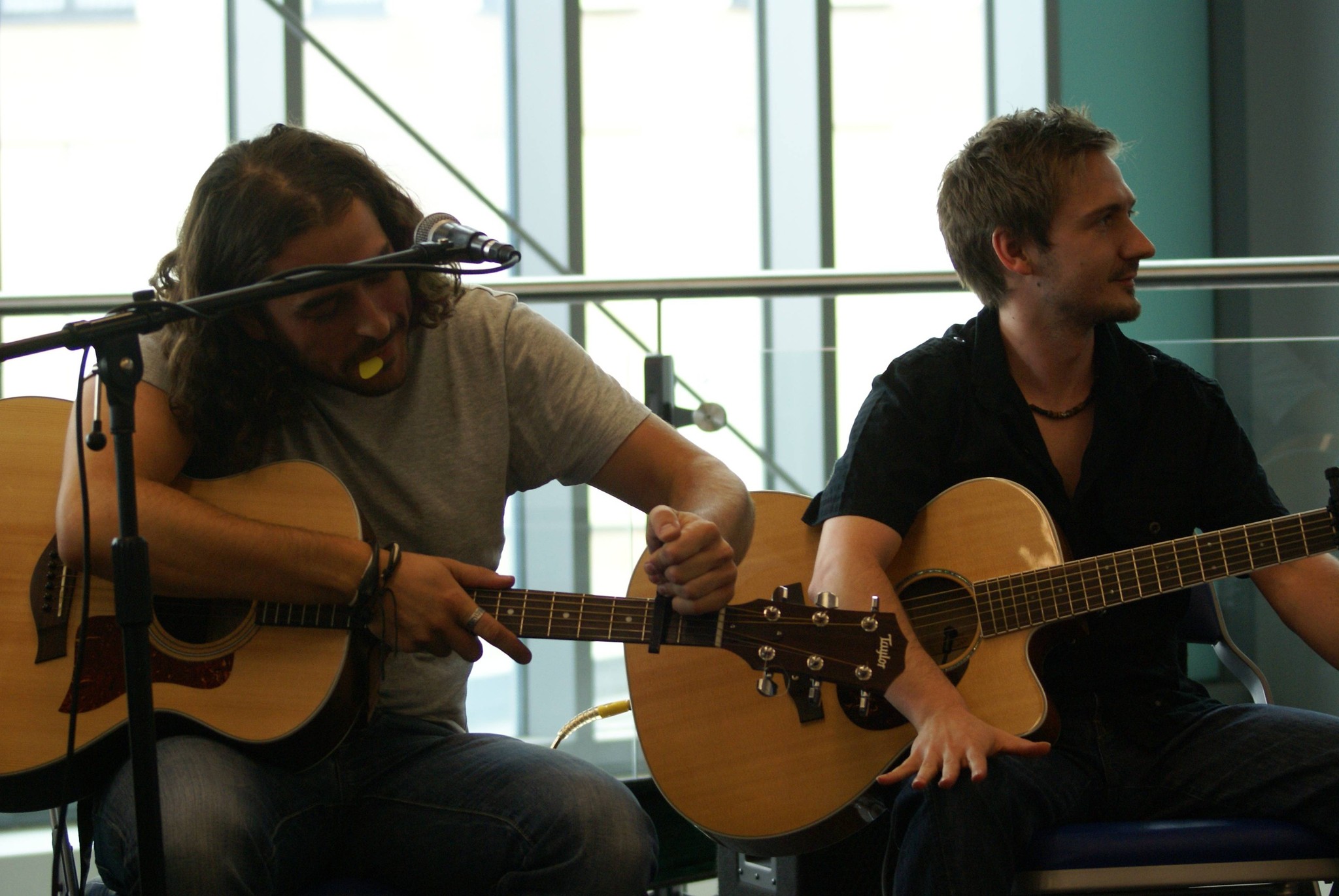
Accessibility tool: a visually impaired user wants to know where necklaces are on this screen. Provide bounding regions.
[1030,333,1103,420]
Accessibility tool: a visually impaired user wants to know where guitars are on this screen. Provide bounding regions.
[1,391,913,816]
[611,467,1332,860]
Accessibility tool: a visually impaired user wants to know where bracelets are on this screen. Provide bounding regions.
[344,539,402,681]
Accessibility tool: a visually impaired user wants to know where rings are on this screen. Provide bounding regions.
[464,607,486,633]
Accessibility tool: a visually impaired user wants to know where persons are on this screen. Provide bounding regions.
[800,104,1339,896]
[55,123,756,896]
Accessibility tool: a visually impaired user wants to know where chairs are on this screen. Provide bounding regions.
[1028,582,1339,896]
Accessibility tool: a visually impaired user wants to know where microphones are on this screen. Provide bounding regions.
[413,212,521,265]
